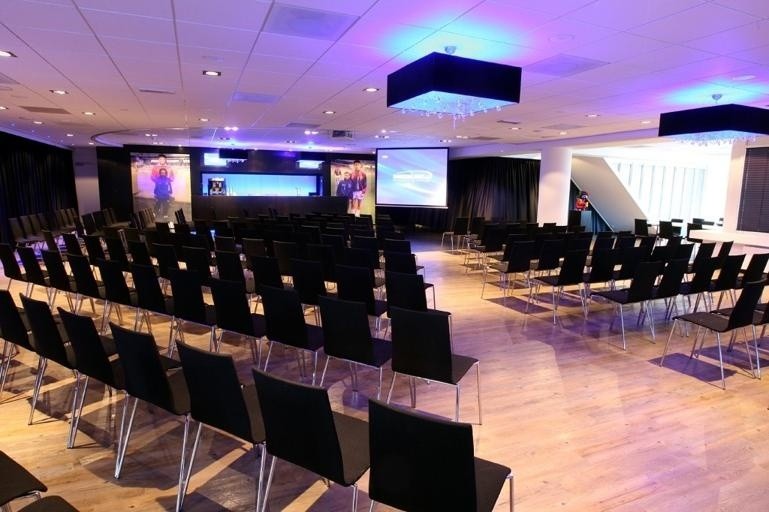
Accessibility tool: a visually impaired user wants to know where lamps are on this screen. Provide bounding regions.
[387,46,522,122]
[658,94,769,147]
[219,144,249,163]
[297,160,324,170]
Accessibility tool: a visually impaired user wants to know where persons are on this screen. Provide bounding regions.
[150,155,174,184]
[574,191,589,211]
[152,168,173,218]
[334,159,367,218]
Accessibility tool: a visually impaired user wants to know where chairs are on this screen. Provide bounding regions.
[251,368,370,512]
[108,321,191,512]
[57,306,182,478]
[19,293,117,449]
[369,397,512,512]
[175,340,266,512]
[385,305,482,424]
